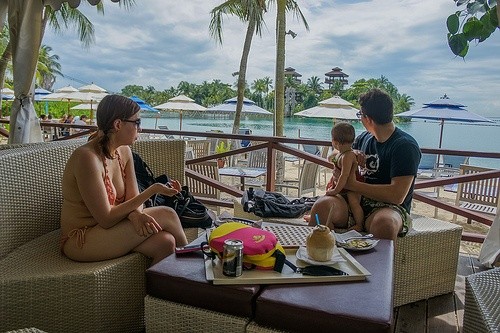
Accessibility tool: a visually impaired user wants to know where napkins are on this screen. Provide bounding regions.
[298,245,346,262]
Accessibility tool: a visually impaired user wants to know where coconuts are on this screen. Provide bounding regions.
[307,225,335,260]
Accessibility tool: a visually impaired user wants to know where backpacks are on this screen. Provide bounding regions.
[241,187,307,218]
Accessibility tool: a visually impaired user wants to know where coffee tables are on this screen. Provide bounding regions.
[144,222,394,333]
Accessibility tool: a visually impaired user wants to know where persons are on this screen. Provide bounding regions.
[38,94,188,264]
[303,88,421,252]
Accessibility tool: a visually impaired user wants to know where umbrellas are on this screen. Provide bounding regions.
[0,81,496,165]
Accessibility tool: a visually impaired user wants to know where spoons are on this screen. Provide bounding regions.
[336,234,374,243]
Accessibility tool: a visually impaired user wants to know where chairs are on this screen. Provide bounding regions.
[453,164,500,223]
[39,120,329,216]
[417,170,433,178]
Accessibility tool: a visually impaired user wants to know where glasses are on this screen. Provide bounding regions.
[122,118,140,126]
[356,110,366,119]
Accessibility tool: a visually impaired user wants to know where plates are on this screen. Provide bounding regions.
[334,228,381,250]
[296,246,348,265]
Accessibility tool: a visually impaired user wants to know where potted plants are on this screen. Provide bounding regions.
[216,141,232,168]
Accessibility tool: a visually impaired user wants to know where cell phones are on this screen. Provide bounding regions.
[175,244,210,254]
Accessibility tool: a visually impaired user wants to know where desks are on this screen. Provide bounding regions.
[219,167,267,191]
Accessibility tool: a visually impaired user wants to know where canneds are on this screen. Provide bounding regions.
[222,240,243,277]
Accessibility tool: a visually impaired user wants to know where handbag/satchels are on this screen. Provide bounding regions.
[200,222,287,273]
[292,195,320,209]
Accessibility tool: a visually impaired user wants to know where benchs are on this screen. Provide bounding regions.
[0,139,204,333]
[234,194,463,307]
[464,266,500,333]
